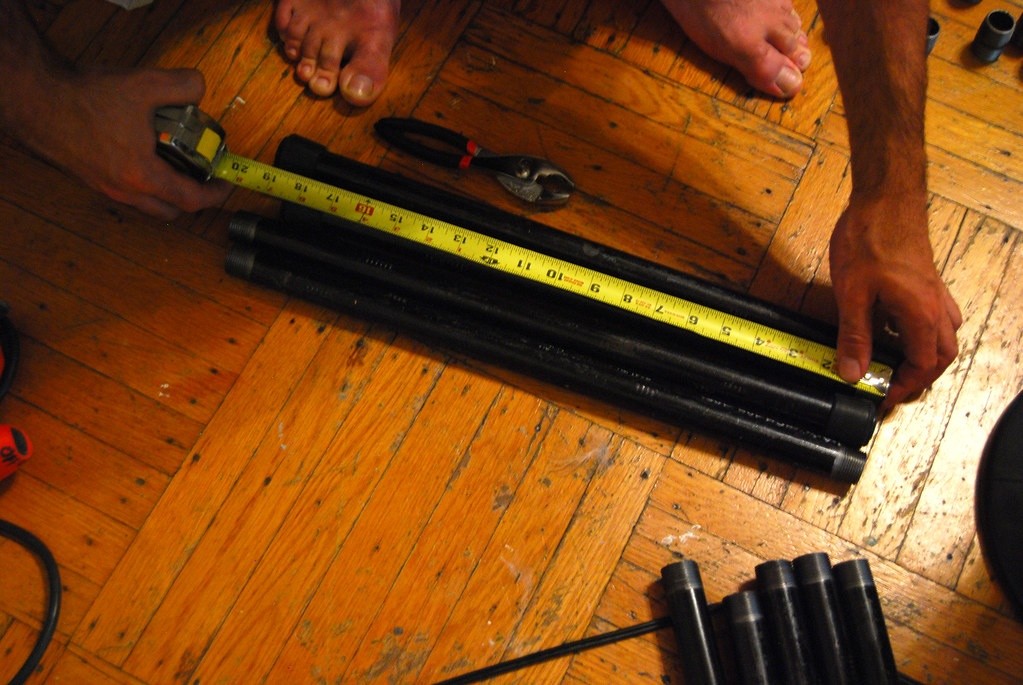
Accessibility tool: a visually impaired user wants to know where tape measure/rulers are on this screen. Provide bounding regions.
[142,101,894,399]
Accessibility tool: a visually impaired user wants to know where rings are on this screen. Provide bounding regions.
[885,320,903,337]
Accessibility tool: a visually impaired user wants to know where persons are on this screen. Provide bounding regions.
[0,0,963,411]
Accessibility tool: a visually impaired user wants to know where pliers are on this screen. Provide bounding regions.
[368,110,578,214]
